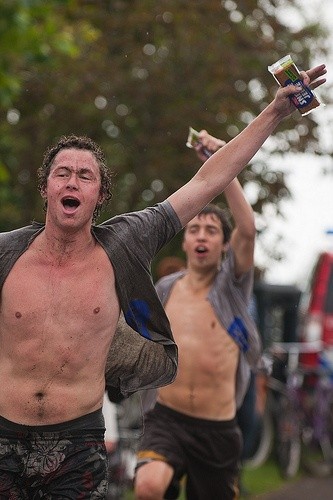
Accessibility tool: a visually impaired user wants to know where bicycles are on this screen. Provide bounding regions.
[238,339,333,482]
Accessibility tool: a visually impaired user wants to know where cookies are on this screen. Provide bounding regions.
[191,133,214,157]
[274,64,320,114]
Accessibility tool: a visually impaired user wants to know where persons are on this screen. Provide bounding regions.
[102,389,121,454]
[0,63,328,500]
[133,128,255,500]
